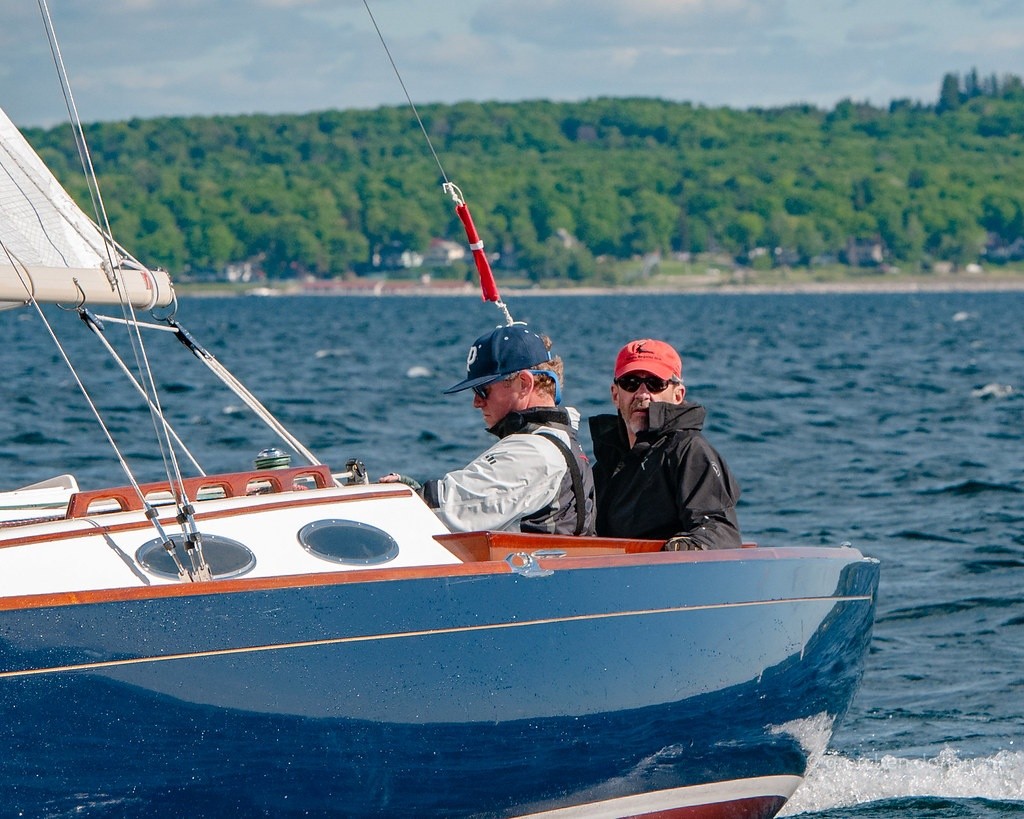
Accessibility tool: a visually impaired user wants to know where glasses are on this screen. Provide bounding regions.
[617,374,678,394]
[472,375,510,400]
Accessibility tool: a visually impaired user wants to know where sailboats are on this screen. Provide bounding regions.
[0,108,885,819]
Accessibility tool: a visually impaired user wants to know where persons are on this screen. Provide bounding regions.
[589,340,742,552]
[379,326,597,537]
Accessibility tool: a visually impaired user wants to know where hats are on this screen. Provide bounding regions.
[614,338,682,380]
[444,328,551,393]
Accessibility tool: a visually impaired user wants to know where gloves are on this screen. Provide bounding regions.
[662,536,703,552]
